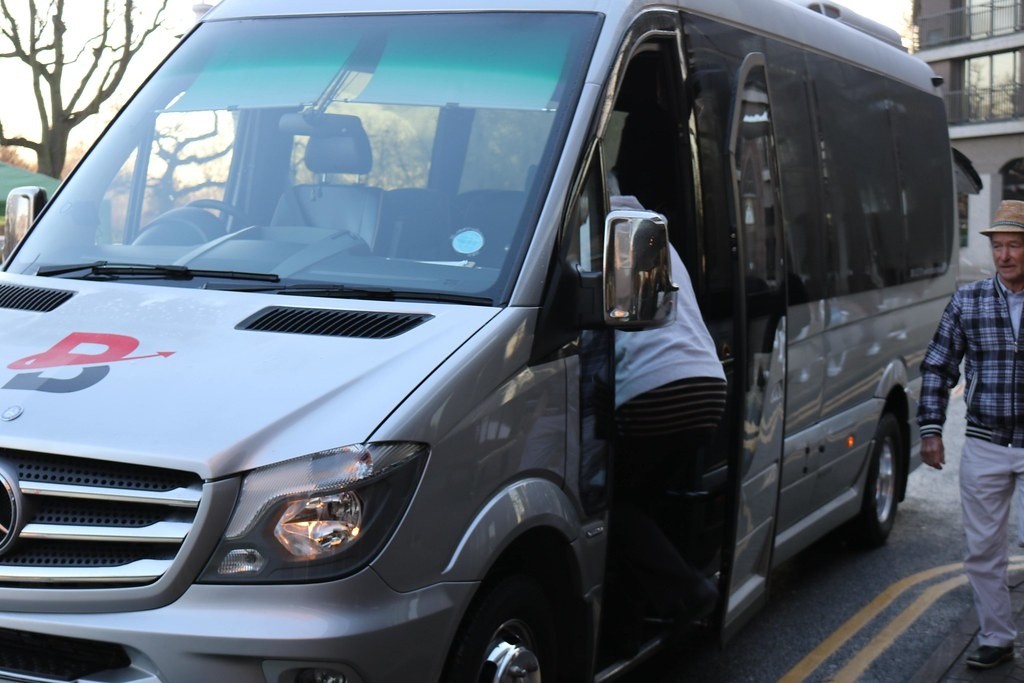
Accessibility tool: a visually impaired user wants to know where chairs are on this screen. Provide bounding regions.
[270,116,385,249]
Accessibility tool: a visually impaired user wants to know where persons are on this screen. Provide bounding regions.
[569,169,729,658]
[914,201,1024,668]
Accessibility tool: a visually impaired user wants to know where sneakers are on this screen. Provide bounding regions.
[966,645,1014,668]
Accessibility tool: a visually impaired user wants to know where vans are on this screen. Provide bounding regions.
[0,0,984,683]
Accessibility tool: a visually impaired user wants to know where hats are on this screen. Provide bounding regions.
[981,200,1024,237]
